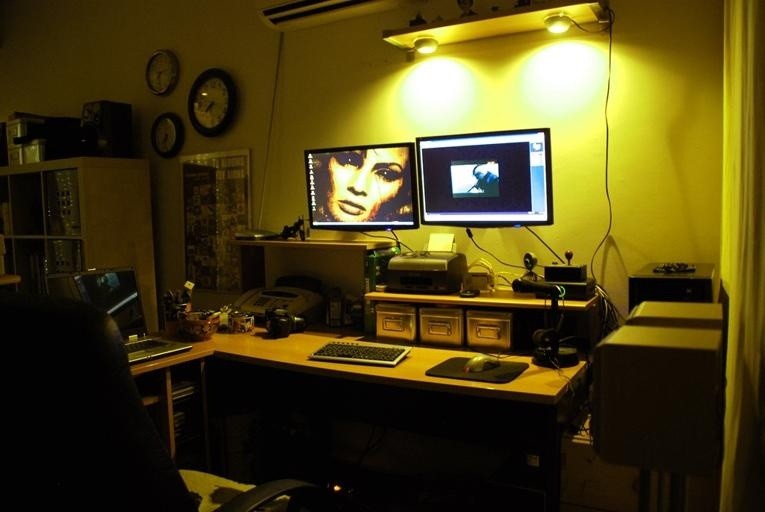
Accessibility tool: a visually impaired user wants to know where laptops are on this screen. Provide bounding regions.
[73,266,193,367]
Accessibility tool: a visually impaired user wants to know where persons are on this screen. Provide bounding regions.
[309,146,413,224]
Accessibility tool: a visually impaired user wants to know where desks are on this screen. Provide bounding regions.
[215,238,602,512]
[121,337,214,472]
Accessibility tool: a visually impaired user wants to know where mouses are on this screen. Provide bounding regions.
[463,353,501,374]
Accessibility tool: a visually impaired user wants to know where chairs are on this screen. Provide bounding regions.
[2,292,327,512]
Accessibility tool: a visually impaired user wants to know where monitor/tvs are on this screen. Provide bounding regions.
[415,128,553,228]
[303,142,420,231]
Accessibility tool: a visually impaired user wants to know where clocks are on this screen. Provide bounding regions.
[188,68,235,136]
[145,49,178,95]
[150,113,182,158]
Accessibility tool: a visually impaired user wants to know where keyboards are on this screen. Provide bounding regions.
[307,341,410,367]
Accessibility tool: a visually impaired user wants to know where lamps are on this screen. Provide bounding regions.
[545,16,571,33]
[415,39,439,54]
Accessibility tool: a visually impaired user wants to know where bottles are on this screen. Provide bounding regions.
[325,287,363,332]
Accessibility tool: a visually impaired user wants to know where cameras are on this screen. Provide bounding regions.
[265,307,305,339]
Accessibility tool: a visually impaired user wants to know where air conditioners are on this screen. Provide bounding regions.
[256,1,411,33]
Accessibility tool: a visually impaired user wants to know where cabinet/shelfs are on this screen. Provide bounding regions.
[0,156,158,338]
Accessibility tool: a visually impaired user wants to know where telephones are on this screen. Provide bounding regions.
[235,287,321,318]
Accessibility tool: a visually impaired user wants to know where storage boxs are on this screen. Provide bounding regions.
[419,307,464,345]
[375,304,417,341]
[7,148,23,165]
[465,310,513,348]
[24,144,46,163]
[6,119,45,147]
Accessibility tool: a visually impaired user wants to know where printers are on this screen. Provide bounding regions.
[388,250,469,295]
